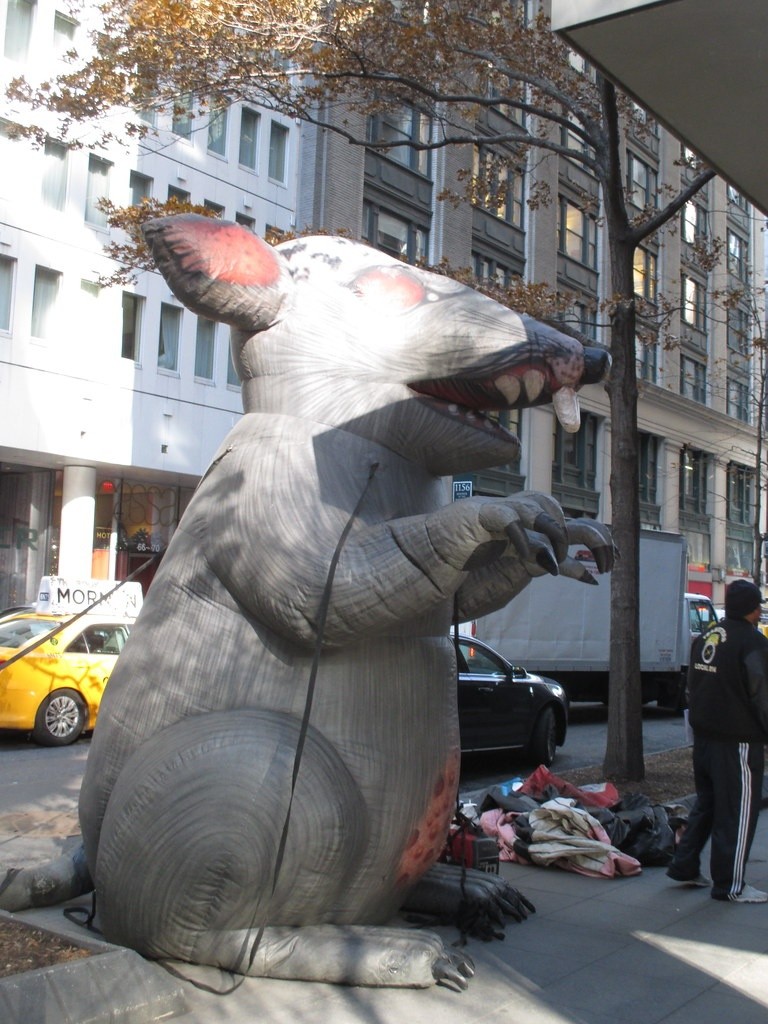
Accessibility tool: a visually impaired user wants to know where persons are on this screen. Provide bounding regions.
[665,579,768,903]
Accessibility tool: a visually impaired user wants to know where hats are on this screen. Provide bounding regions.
[724,580,762,618]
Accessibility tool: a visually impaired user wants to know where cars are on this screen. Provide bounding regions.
[1,576,146,747]
[449,632,571,768]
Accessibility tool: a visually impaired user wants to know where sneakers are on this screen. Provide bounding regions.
[665,868,713,887]
[734,884,768,902]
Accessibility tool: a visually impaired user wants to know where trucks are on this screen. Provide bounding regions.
[449,517,719,712]
